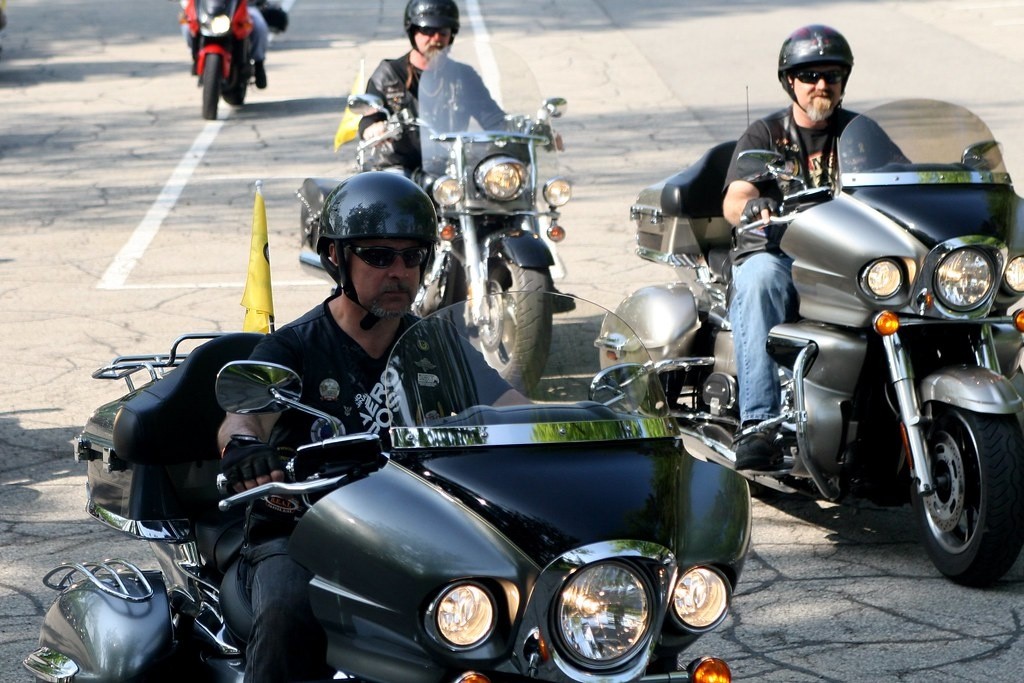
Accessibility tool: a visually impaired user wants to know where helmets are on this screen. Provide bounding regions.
[316,171,438,256]
[777,24,853,79]
[404,0,460,37]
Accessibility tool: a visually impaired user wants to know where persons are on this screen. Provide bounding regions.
[359,0,563,179]
[722,24,913,470]
[217,172,536,683]
[182,0,268,88]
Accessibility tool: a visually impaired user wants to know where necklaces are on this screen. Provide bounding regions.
[412,65,444,97]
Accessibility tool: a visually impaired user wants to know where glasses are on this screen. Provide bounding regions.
[786,70,848,85]
[341,238,433,270]
[416,25,450,37]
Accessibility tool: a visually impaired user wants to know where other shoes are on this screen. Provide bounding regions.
[550,286,574,313]
[252,62,267,89]
[732,423,786,477]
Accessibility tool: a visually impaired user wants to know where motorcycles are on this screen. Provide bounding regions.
[181,0,257,120]
[299,38,577,396]
[593,99,1023,587]
[24,291,753,683]
